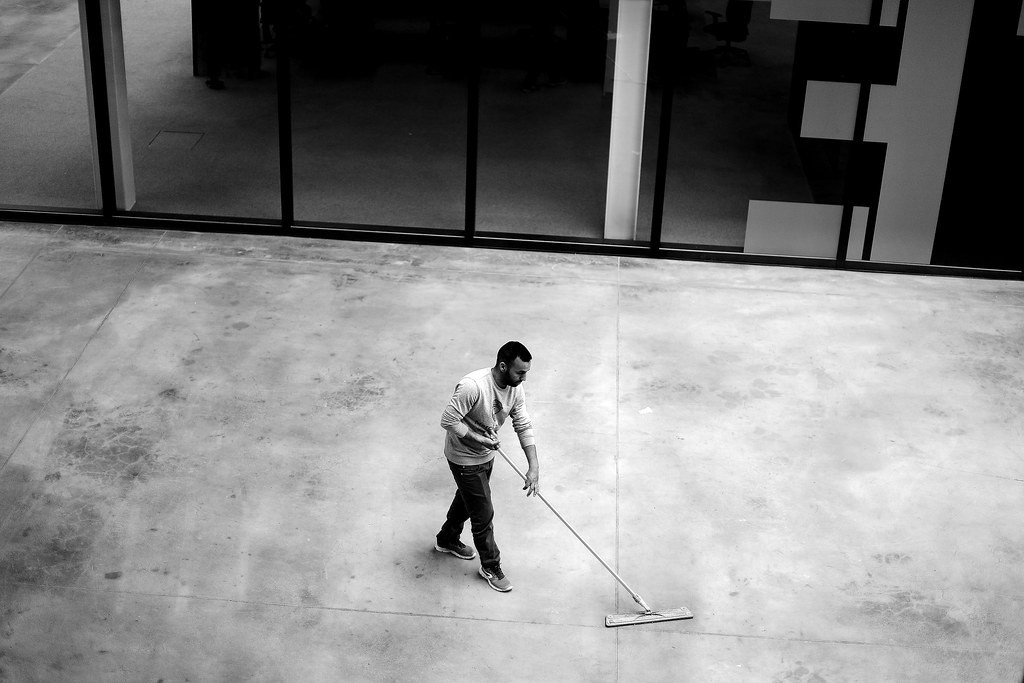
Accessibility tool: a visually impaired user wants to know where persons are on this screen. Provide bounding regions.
[433,341,542,592]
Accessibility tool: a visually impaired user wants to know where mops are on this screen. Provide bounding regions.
[464,415,694,629]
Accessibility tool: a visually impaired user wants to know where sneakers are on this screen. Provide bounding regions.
[479,565,513,592]
[436,538,476,560]
